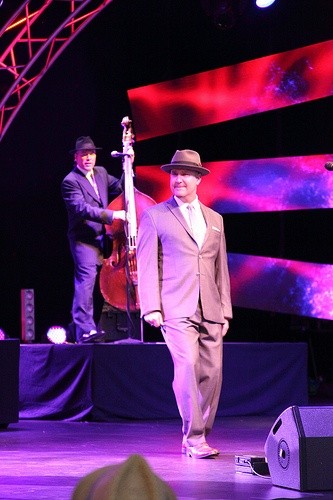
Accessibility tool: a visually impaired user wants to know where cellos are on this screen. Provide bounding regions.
[100,116,158,342]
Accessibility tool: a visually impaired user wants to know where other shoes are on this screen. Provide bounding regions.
[77,329,105,344]
[182,441,220,456]
[186,442,217,460]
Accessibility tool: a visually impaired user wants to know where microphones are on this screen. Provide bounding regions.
[325,162,333,170]
[112,150,132,157]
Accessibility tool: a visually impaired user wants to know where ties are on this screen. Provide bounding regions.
[88,173,100,199]
[187,205,202,249]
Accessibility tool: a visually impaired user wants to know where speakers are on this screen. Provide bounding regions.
[20,288,35,342]
[0,340,20,427]
[263,405,333,491]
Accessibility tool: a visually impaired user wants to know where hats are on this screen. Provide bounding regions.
[161,150,210,176]
[72,135,101,151]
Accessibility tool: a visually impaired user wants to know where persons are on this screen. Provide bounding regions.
[62,136,135,344]
[136,150,232,458]
